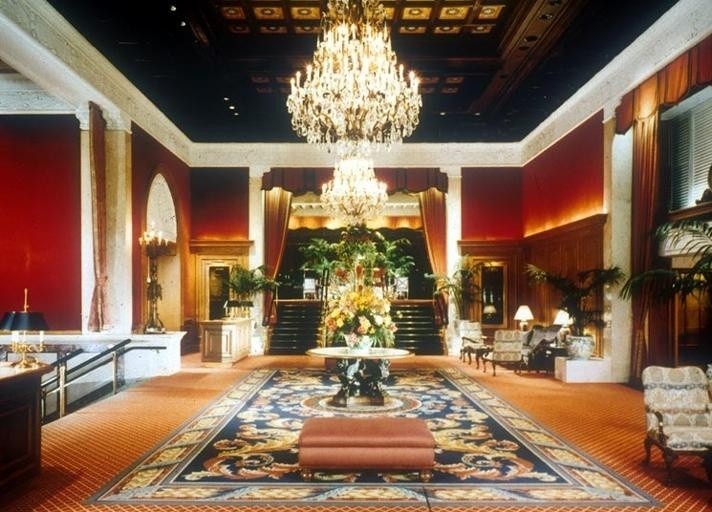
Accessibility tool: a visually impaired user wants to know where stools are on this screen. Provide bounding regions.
[297,417,435,482]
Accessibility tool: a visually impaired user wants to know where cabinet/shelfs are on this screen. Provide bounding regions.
[0,366,43,507]
[198,317,253,363]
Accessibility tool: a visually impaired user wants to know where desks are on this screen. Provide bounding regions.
[307,346,413,409]
[467,344,493,368]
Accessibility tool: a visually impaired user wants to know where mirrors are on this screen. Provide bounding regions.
[479,261,509,329]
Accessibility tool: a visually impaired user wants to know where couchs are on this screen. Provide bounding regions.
[524,324,564,373]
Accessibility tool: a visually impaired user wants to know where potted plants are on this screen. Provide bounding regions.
[525,263,625,360]
[425,254,483,359]
[617,218,712,396]
[221,263,280,357]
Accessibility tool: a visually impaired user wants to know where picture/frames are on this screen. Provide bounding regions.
[347,359,360,397]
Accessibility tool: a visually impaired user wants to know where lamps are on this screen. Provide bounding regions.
[0,289,53,369]
[137,230,168,334]
[514,305,533,329]
[483,305,497,323]
[287,1,423,217]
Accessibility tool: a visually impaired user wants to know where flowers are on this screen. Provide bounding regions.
[319,288,398,350]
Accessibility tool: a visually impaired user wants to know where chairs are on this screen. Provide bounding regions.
[459,336,478,363]
[483,330,524,377]
[642,365,712,488]
[529,328,567,375]
